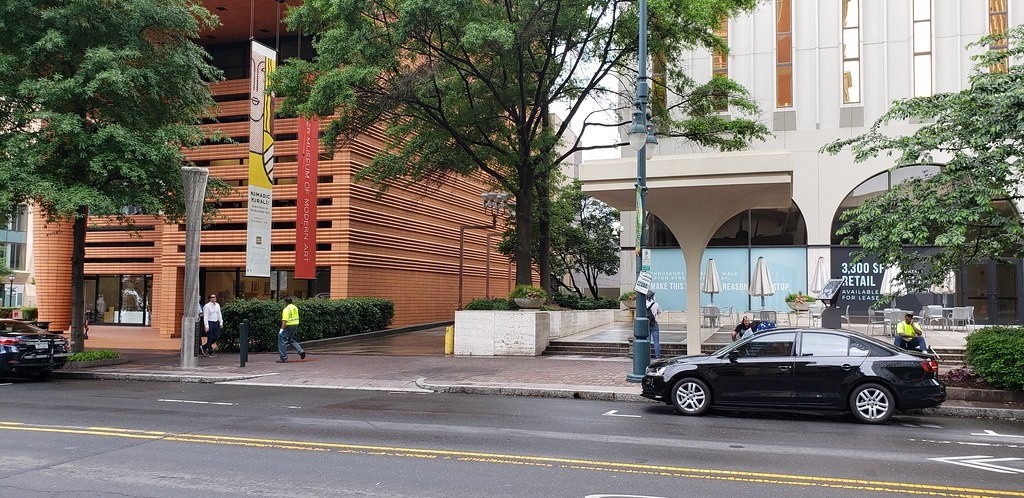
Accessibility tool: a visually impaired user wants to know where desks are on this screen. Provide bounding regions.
[744,311,761,323]
[942,307,954,329]
[876,310,893,336]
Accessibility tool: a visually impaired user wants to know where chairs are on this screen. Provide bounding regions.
[701,305,975,339]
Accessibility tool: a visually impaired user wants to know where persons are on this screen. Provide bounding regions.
[646,289,662,360]
[198,294,223,358]
[276,297,306,363]
[894,313,928,355]
[731,316,751,342]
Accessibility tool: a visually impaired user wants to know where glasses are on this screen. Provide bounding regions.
[211,297,217,298]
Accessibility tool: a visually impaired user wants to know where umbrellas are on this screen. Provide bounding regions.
[701,257,722,304]
[930,250,956,308]
[748,257,774,311]
[880,252,907,310]
[810,256,828,306]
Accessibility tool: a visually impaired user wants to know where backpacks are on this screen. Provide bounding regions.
[647,302,656,327]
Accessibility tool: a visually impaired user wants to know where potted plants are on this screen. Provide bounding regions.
[785,293,816,310]
[619,291,637,308]
[510,284,547,308]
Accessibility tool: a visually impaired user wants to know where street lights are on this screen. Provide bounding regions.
[8,273,15,308]
[627,98,659,383]
[458,190,511,309]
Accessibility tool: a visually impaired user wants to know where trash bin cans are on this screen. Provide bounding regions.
[26,321,52,331]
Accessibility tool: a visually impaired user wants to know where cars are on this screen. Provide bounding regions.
[0,319,68,380]
[639,326,948,421]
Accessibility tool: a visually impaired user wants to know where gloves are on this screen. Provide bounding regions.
[279,328,284,337]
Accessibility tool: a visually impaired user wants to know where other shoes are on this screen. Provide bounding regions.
[923,350,927,354]
[300,351,305,359]
[276,358,288,363]
[209,353,217,357]
[200,345,207,356]
